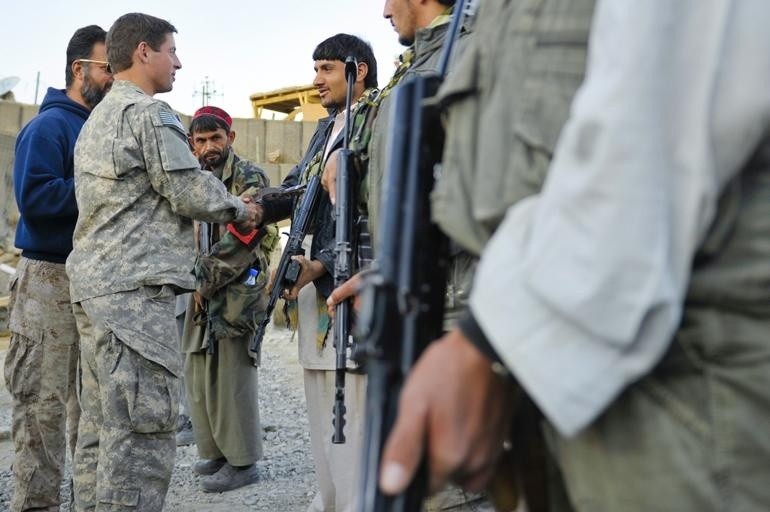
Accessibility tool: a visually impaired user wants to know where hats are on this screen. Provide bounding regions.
[191,107,232,128]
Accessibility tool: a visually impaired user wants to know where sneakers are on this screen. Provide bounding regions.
[194,456,227,474]
[198,463,259,491]
[175,421,196,445]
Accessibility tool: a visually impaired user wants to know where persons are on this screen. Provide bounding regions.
[4,25,115,511]
[242,32,379,510]
[321,0,770,511]
[175,106,280,492]
[66,13,257,511]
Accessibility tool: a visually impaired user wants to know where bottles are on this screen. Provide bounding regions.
[240,269,258,285]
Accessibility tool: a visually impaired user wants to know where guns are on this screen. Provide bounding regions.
[332,55,367,442]
[251,174,330,353]
[349,1,471,512]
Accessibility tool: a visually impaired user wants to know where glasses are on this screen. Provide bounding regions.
[80,59,112,73]
